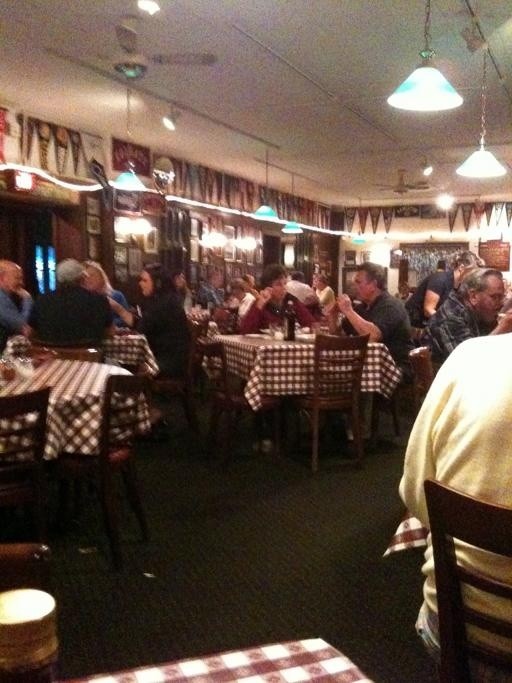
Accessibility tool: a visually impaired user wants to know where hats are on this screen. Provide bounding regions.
[55,258,90,282]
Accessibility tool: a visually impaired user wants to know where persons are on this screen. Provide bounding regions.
[0,249,512,456]
[396,331,512,683]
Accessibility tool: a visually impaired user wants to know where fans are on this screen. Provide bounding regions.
[56,14,220,79]
[370,166,430,195]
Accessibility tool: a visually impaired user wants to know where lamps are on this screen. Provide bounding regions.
[134,1,160,16]
[384,0,465,114]
[281,171,305,234]
[158,104,176,129]
[255,144,278,218]
[456,44,507,180]
[416,153,432,178]
[108,82,146,193]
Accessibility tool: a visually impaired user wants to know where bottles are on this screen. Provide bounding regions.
[283,299,297,341]
